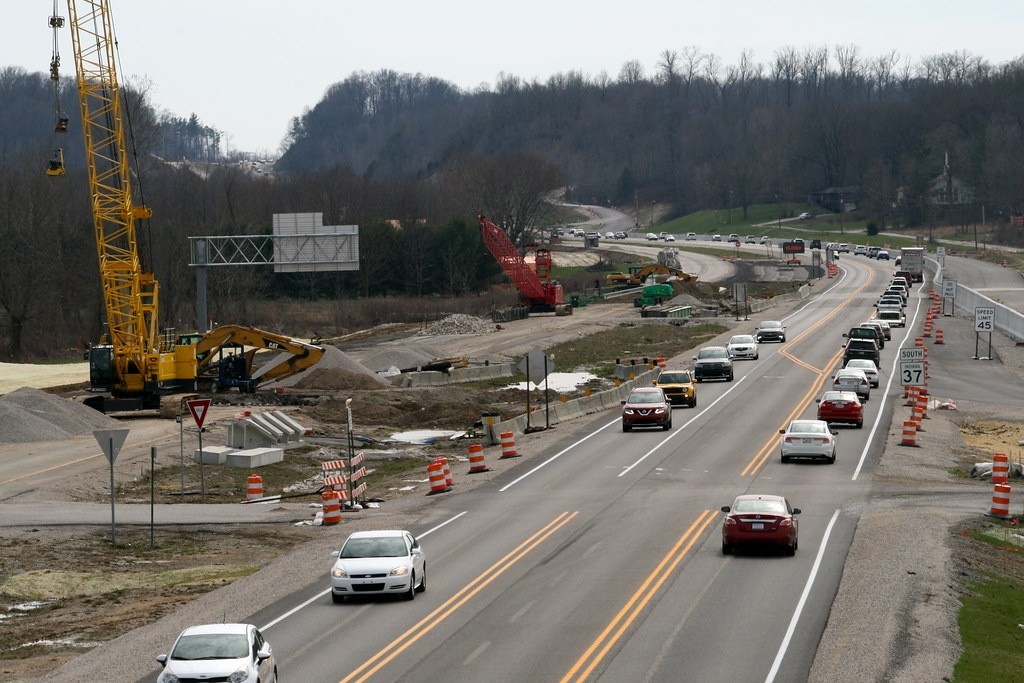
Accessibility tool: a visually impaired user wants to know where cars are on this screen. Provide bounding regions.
[331,530,426,602]
[755,321,786,343]
[794,239,901,266]
[156,622,277,683]
[873,271,911,327]
[832,319,891,400]
[721,494,802,556]
[815,391,864,428]
[557,229,771,244]
[779,420,839,464]
[726,335,759,360]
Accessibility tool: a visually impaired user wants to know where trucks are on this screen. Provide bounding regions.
[901,248,925,282]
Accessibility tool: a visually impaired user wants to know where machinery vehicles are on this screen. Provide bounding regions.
[478,214,574,316]
[46,0,324,419]
[607,265,698,292]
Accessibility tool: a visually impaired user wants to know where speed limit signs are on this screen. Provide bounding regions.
[975,307,994,333]
[900,361,925,386]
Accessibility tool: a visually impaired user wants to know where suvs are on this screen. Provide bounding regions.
[653,370,697,408]
[621,385,672,432]
[692,346,734,382]
